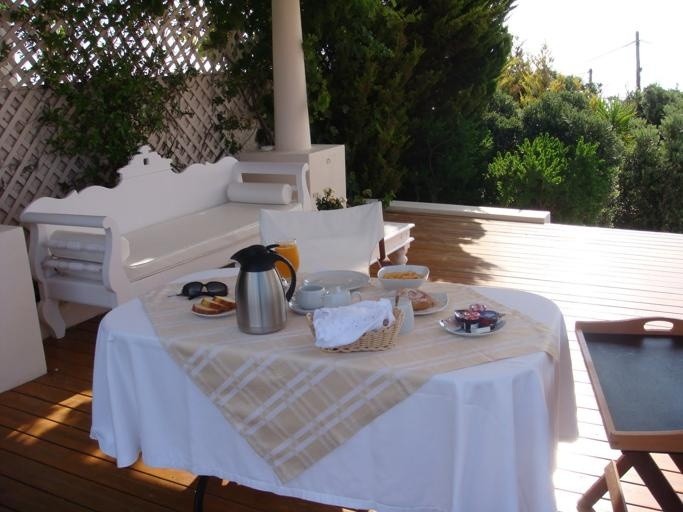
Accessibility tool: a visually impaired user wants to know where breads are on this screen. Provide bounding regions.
[192,296,236,314]
[396,287,432,310]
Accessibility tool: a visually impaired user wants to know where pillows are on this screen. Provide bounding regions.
[48,229,130,264]
[225,181,294,206]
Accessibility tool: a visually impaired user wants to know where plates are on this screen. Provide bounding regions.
[303,270,370,292]
[190,296,235,317]
[381,292,506,336]
[289,298,318,315]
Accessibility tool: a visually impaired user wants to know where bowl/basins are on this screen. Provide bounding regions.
[377,265,430,290]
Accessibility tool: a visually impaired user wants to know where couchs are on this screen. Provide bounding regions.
[21,141,317,338]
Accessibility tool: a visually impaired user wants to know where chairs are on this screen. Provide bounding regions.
[256,203,388,279]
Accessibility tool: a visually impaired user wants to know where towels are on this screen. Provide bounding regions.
[312,297,396,349]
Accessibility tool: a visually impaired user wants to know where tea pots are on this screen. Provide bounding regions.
[230,245,295,335]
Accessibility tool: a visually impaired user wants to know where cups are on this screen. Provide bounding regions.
[271,238,301,280]
[297,283,363,309]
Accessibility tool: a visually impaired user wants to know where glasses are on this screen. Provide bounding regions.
[181,282,229,300]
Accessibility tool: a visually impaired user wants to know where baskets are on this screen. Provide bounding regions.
[306,306,404,352]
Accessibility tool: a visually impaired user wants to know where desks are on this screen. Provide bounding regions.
[0,223,50,392]
[572,315,682,511]
